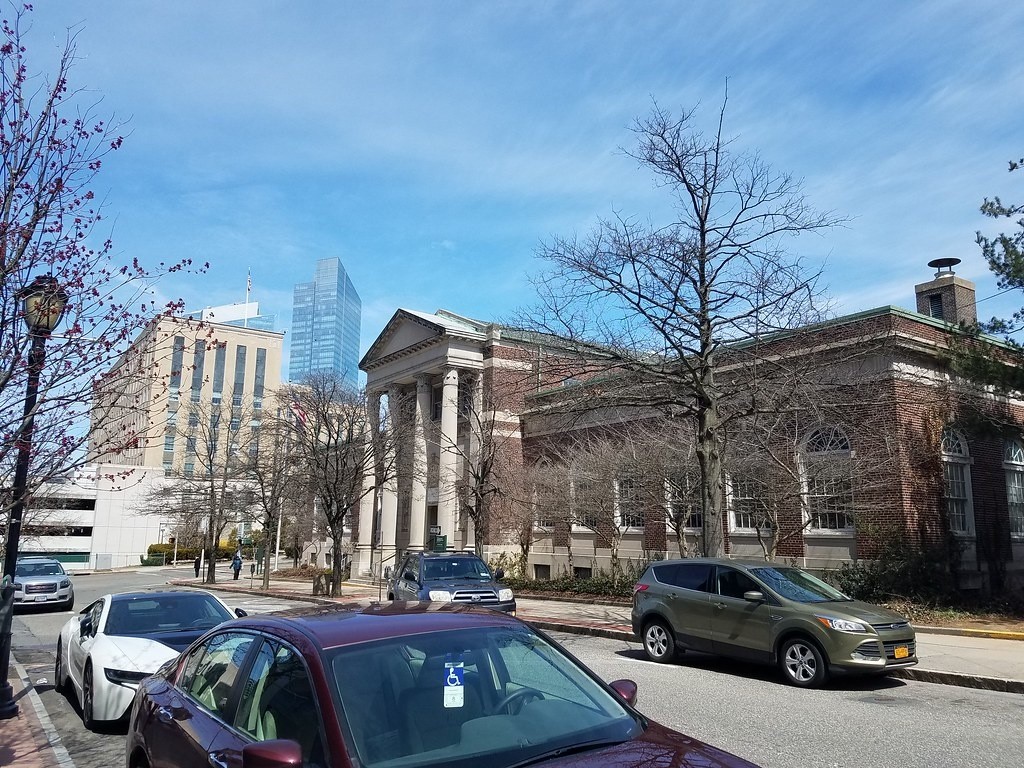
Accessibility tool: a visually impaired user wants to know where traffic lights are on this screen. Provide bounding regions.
[169,538,175,544]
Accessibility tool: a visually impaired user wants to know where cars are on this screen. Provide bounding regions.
[125,597,765,768]
[53,588,248,729]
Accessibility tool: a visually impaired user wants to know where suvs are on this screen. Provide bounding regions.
[387,550,517,618]
[13,557,76,611]
[632,559,918,683]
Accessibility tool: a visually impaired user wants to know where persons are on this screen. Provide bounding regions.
[229,556,244,581]
[194,556,201,578]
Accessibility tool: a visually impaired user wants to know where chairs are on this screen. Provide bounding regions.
[400,657,489,752]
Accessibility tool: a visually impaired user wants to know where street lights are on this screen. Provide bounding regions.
[1,276,68,719]
